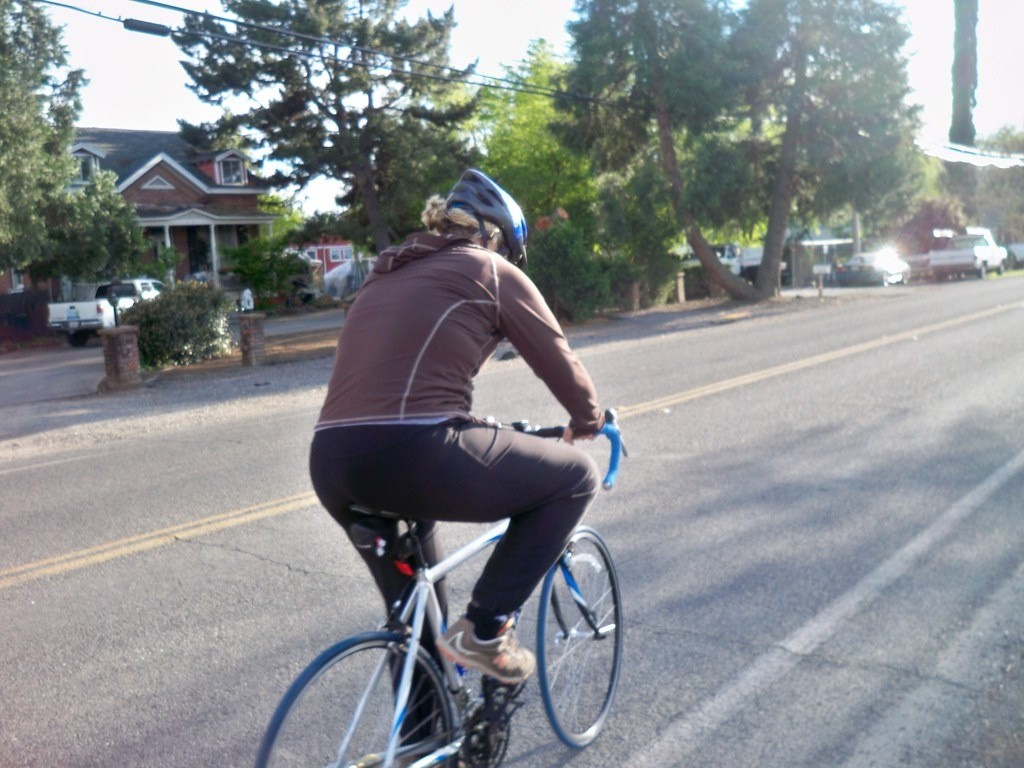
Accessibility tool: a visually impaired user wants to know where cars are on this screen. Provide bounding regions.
[1003,242,1024,270]
[834,251,912,287]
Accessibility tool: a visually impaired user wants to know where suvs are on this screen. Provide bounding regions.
[193,271,255,316]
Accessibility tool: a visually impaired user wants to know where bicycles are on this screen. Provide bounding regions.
[253,409,627,767]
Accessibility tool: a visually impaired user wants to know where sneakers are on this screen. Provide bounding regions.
[433,616,537,685]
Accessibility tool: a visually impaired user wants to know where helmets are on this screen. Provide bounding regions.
[446,170,531,270]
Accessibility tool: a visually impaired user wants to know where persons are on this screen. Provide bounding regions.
[309,168,605,768]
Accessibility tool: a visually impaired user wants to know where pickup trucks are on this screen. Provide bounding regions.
[928,234,1008,282]
[45,276,166,349]
